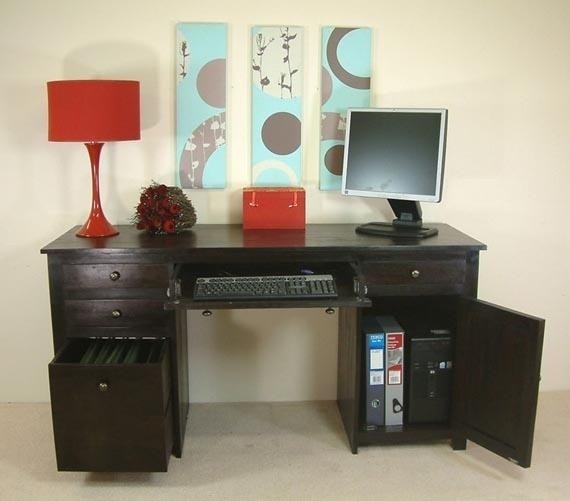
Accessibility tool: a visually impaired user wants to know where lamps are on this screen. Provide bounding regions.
[46,81,142,239]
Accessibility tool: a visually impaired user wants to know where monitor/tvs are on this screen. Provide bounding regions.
[341,106,449,238]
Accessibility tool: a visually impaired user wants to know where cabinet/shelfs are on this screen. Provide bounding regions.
[39,222,546,472]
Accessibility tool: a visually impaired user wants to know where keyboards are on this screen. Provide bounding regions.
[193,270,339,300]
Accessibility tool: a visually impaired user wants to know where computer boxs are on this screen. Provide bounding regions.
[398,316,454,425]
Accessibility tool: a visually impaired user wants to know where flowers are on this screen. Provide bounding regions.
[128,181,197,236]
